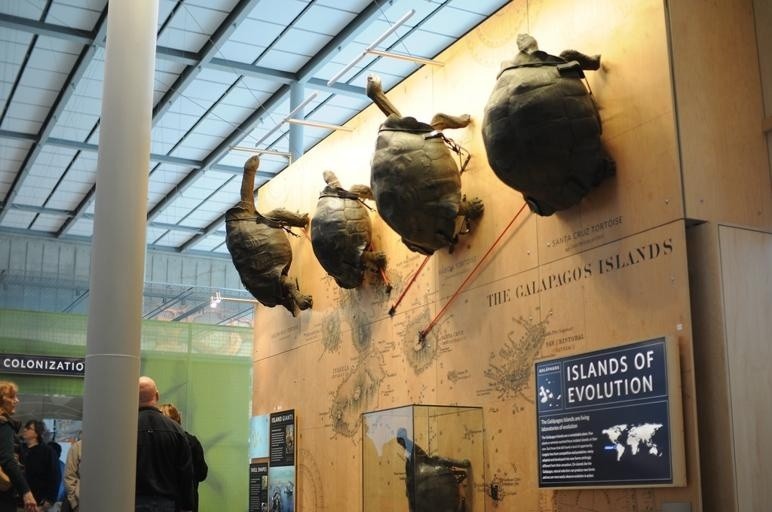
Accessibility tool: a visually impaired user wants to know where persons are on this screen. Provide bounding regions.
[135,375,195,512]
[159,402,209,512]
[0,381,39,512]
[48,440,67,511]
[65,439,80,512]
[18,420,62,511]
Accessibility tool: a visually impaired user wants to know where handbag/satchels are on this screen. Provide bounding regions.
[0,453,19,492]
